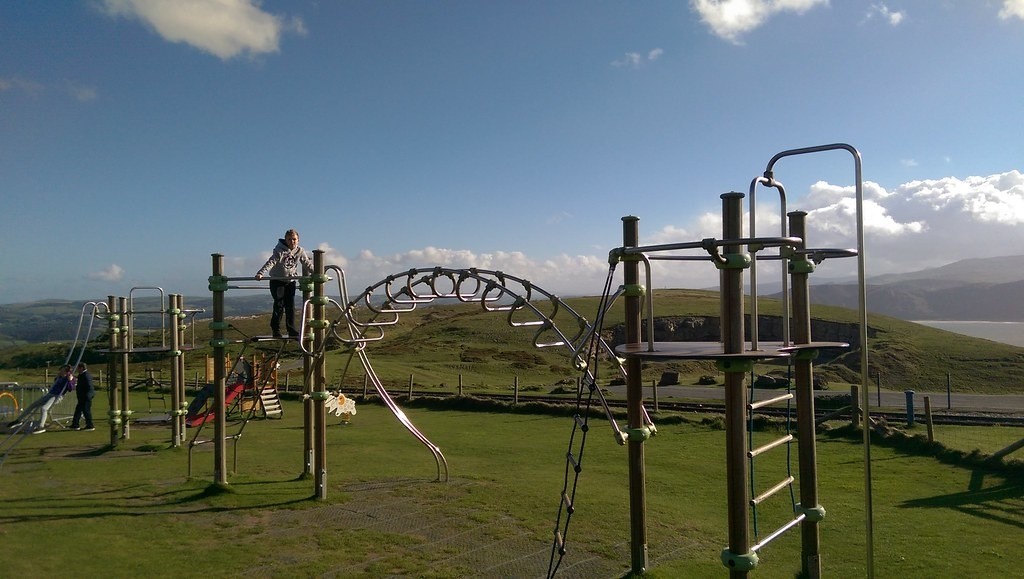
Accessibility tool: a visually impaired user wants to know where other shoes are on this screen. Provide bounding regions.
[32,426,46,435]
[286,327,299,336]
[65,423,80,431]
[81,425,95,431]
[7,419,23,429]
[272,331,283,338]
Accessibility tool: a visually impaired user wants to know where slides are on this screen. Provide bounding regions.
[185,382,254,428]
[185,384,214,420]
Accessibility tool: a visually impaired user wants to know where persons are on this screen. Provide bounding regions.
[255,229,314,338]
[8,364,78,435]
[68,362,96,431]
